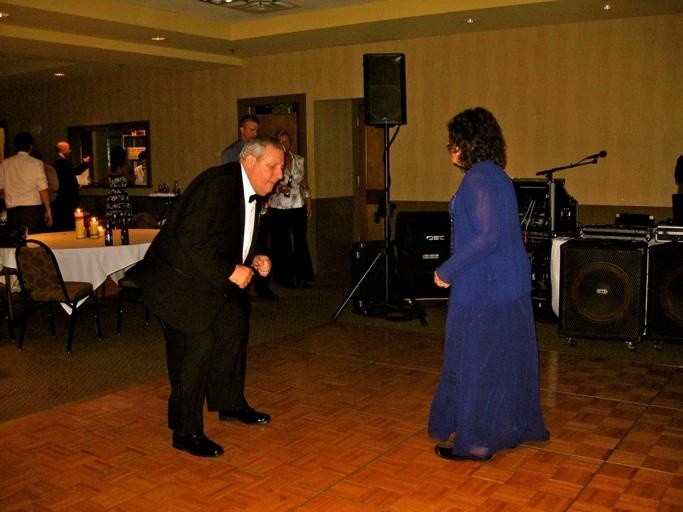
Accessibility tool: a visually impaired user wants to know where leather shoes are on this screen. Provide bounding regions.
[435,443,495,460]
[173,432,224,457]
[220,408,271,425]
[257,293,279,300]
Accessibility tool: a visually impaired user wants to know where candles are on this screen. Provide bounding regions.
[89,216,99,238]
[74,208,85,239]
[100,226,105,237]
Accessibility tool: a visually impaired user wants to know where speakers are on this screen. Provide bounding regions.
[397,210,451,301]
[354,243,398,310]
[363,52,407,126]
[561,239,646,343]
[647,244,682,341]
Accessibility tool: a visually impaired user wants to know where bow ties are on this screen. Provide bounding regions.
[249,195,260,203]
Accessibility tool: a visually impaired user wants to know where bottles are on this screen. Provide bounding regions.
[172,180,180,195]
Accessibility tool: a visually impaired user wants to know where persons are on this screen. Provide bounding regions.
[134,150,148,187]
[269,130,313,289]
[428,106,550,461]
[105,145,136,228]
[221,115,280,301]
[136,135,286,458]
[0,132,90,231]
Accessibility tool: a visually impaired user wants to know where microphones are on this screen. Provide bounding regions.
[587,150,608,159]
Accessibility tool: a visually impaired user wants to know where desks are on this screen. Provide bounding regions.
[0,229,169,336]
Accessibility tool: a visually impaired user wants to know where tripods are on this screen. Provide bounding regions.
[328,127,428,327]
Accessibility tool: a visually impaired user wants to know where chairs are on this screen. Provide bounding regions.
[116,213,167,335]
[0,223,28,342]
[14,239,103,355]
